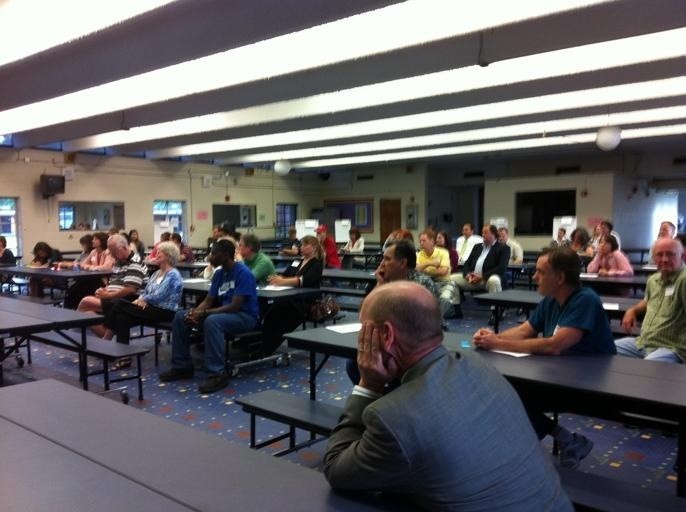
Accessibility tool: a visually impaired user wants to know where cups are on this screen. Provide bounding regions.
[52,262,58,270]
[16,260,23,270]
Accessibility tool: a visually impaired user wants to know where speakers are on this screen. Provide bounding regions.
[40,174,66,194]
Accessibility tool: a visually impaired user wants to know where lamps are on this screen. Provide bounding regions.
[597,104,622,150]
[273,160,292,177]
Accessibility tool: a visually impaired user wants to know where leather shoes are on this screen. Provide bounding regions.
[487,311,495,325]
[444,311,464,319]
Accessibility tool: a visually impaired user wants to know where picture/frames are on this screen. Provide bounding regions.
[406,203,419,229]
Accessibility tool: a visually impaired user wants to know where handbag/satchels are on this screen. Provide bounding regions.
[310,293,340,322]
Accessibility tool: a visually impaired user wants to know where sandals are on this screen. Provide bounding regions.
[110,358,132,371]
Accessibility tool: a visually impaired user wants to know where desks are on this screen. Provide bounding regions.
[0,239,685,511]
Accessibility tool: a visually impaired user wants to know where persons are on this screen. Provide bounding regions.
[145,232,171,279]
[563,227,594,273]
[497,225,523,290]
[473,247,618,479]
[63,232,115,310]
[158,239,260,392]
[415,229,456,332]
[67,234,95,270]
[106,228,119,237]
[337,227,365,286]
[313,224,341,287]
[30,241,63,298]
[345,238,442,388]
[436,230,459,274]
[589,222,600,245]
[238,236,326,374]
[613,238,685,363]
[1,235,16,284]
[548,227,572,249]
[593,221,621,252]
[77,232,151,339]
[322,280,575,511]
[455,222,483,271]
[213,225,239,248]
[387,229,414,241]
[101,242,182,371]
[234,234,276,286]
[278,228,301,268]
[443,224,511,330]
[587,236,635,298]
[648,222,676,265]
[129,230,145,258]
[120,232,138,252]
[170,233,194,310]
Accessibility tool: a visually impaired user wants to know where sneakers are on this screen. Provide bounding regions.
[557,432,594,471]
[159,366,194,382]
[199,375,229,394]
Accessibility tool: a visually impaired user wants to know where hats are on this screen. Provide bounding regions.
[315,225,327,232]
